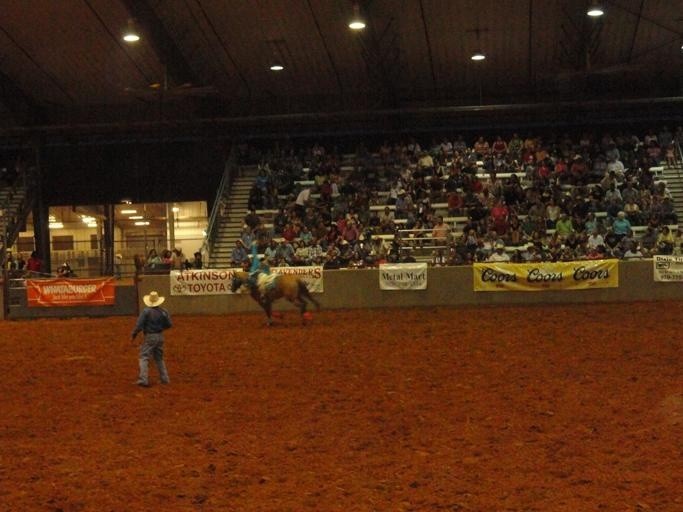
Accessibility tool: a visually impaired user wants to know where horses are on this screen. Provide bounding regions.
[228,269,321,327]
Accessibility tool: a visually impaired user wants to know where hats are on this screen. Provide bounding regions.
[142,291,165,308]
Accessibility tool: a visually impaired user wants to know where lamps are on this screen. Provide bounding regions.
[122,17,140,44]
[469,39,486,61]
[586,1,604,18]
[346,2,365,31]
[268,48,284,71]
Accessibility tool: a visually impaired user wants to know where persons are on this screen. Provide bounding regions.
[56,262,76,277]
[134,247,202,272]
[9,250,24,271]
[129,290,172,386]
[24,252,44,275]
[230,131,682,275]
